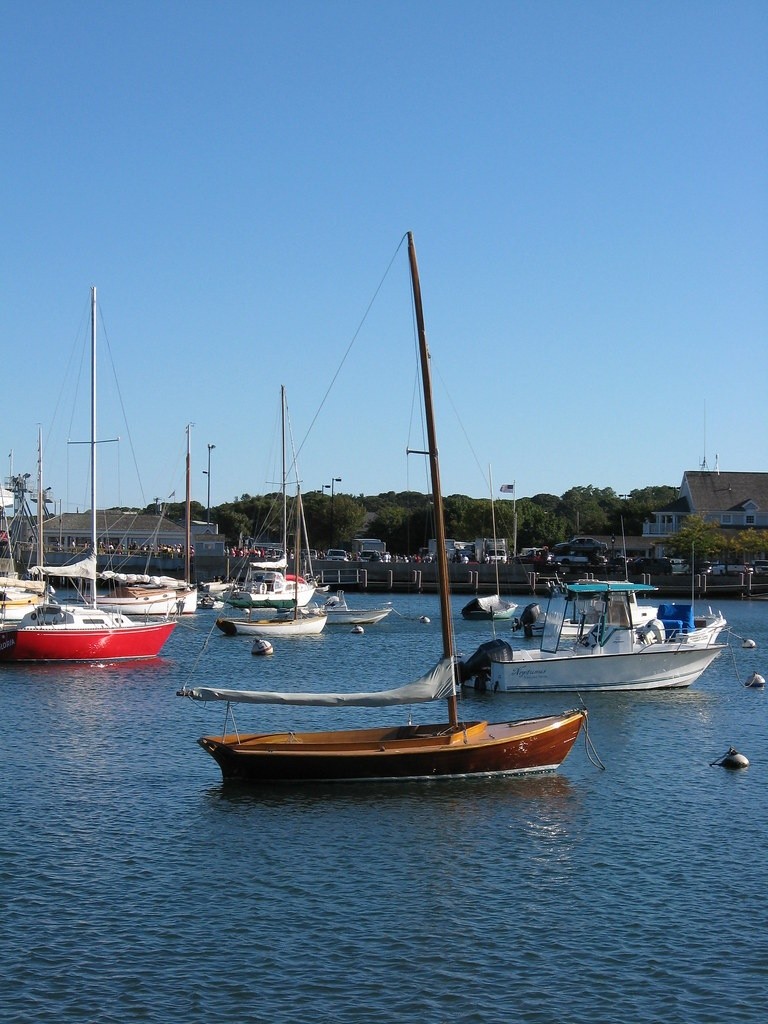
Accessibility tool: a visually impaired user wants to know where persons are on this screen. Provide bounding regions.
[224,544,493,563]
[0,531,194,557]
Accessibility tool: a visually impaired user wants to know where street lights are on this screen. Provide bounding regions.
[329,477,343,549]
[321,485,330,498]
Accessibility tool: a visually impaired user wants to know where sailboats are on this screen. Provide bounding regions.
[300,589,393,627]
[460,462,519,620]
[0,286,327,662]
[176,230,591,786]
[565,516,660,599]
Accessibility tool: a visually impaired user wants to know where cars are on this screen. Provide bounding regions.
[608,551,768,577]
[264,549,555,575]
[552,537,608,557]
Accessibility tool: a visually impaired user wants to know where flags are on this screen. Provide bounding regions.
[500,484,514,494]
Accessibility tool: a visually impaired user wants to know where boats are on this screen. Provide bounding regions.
[452,586,727,691]
[508,579,728,644]
